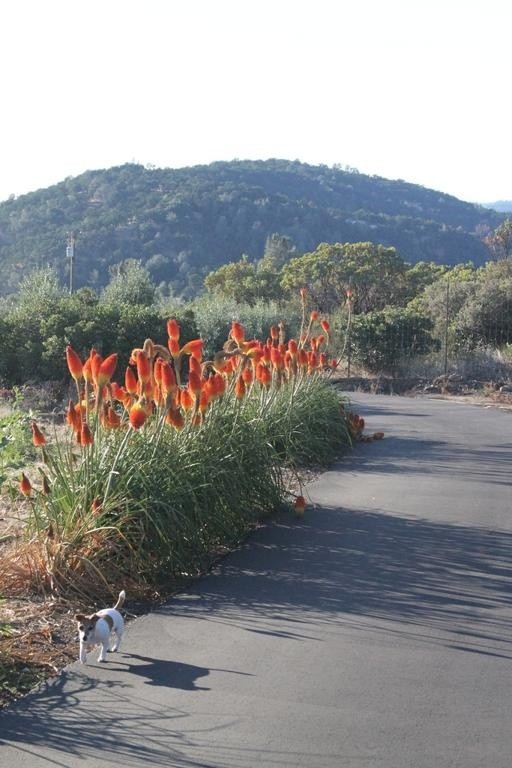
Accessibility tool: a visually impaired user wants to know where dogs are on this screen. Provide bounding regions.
[75,590,127,663]
